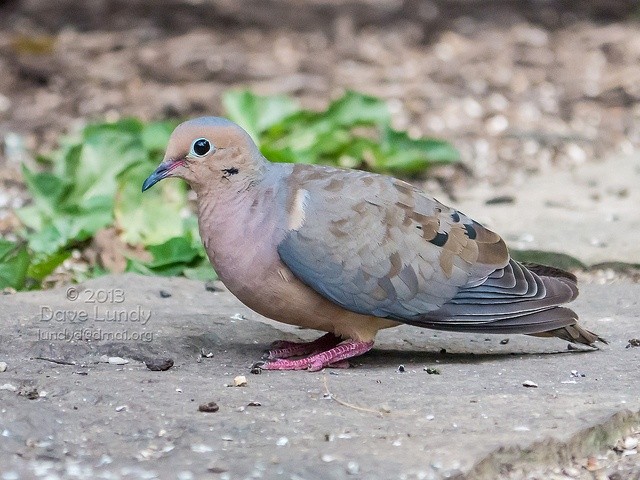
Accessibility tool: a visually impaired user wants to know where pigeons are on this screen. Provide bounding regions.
[142,116,608,371]
